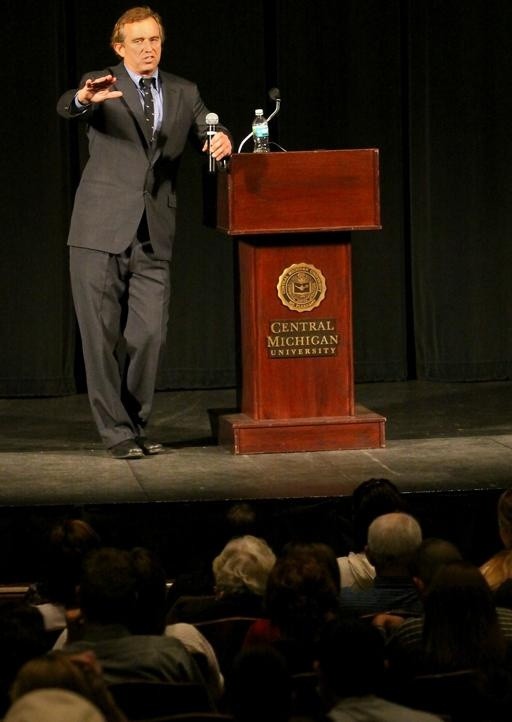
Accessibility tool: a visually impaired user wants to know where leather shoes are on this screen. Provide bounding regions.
[138,437,164,453]
[109,440,145,459]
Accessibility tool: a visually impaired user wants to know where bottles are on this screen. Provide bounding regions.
[252,107,270,154]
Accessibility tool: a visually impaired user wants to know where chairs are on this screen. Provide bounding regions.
[0,562,512,722]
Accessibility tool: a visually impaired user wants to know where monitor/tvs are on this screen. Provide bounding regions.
[268,141,288,152]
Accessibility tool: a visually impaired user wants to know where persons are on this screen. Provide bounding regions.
[1,479,511,722]
[57,7,232,460]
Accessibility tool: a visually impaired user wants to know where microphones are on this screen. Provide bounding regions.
[238,84,283,154]
[204,112,218,174]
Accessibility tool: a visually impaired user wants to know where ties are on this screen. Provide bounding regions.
[140,78,154,144]
